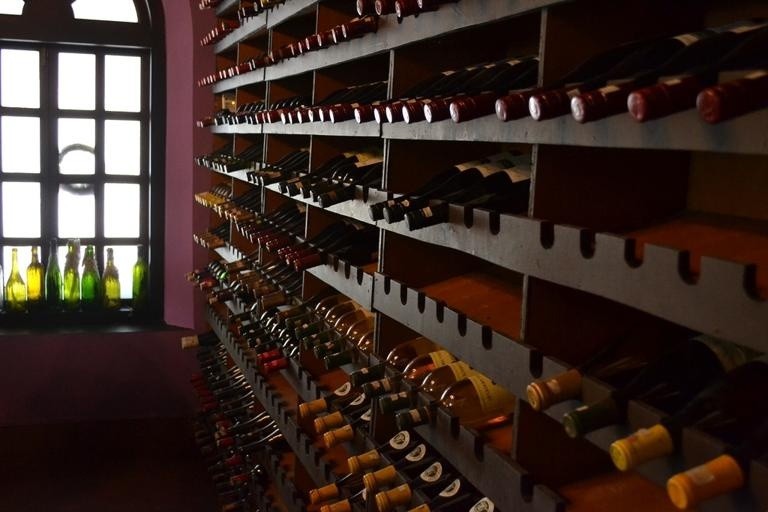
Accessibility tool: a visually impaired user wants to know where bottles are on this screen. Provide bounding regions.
[180,0,768,512]
[4,235,151,328]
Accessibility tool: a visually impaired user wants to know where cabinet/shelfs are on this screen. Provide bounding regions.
[207,0,768,510]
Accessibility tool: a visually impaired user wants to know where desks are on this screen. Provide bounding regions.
[0,321,199,424]
